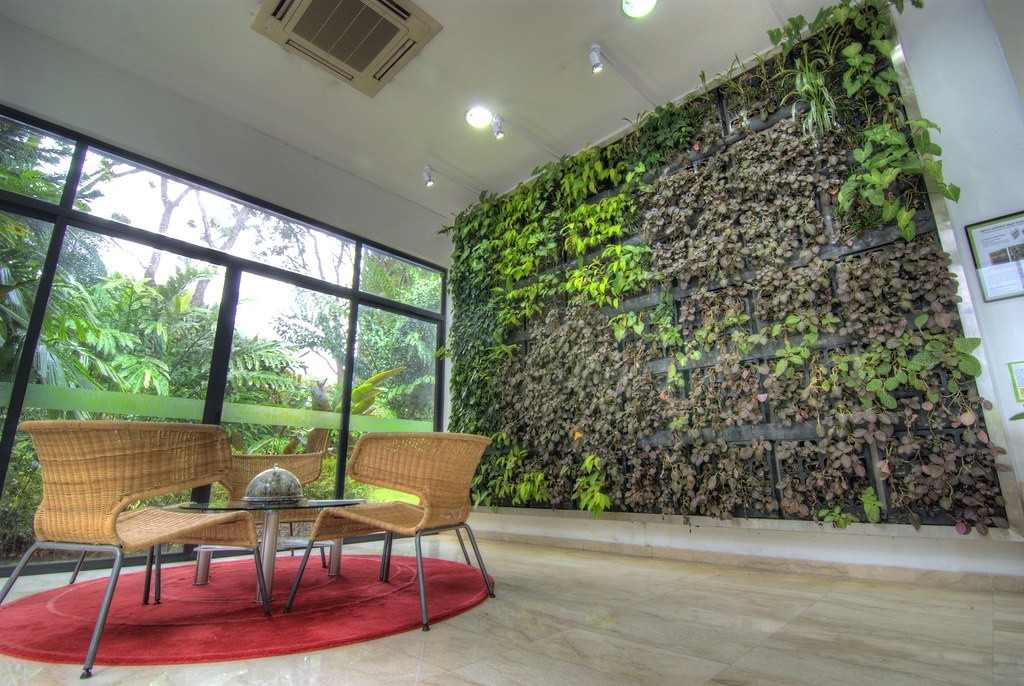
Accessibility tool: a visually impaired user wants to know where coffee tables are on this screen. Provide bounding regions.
[177,500,364,586]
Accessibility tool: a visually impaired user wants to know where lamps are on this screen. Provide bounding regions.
[491,114,505,140]
[589,45,605,73]
[422,166,435,187]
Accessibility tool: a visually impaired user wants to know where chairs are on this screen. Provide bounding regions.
[205,451,330,576]
[0,421,271,679]
[278,433,496,631]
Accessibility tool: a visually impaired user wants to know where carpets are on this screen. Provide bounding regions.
[0,555,494,667]
